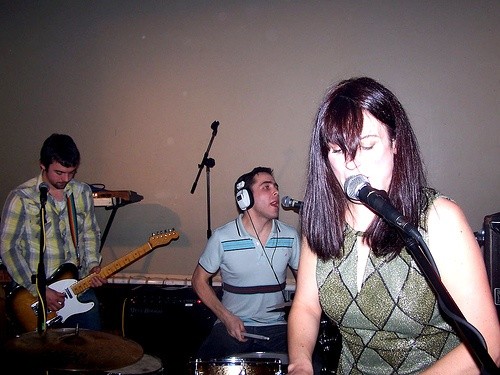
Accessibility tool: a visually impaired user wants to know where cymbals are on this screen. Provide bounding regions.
[6,328,146,373]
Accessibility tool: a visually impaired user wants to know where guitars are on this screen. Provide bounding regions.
[4,228,181,335]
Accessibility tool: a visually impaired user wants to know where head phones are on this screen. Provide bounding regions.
[234,174,254,211]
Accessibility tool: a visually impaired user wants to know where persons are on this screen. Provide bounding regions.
[288,77,500,375]
[0,133,107,332]
[192,167,300,362]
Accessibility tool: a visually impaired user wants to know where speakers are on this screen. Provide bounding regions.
[484,212,500,327]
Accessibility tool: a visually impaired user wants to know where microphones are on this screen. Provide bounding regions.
[281,196,305,209]
[39,182,49,206]
[344,175,421,238]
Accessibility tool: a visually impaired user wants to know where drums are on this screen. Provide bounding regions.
[44,353,164,375]
[219,352,290,375]
[184,357,282,375]
